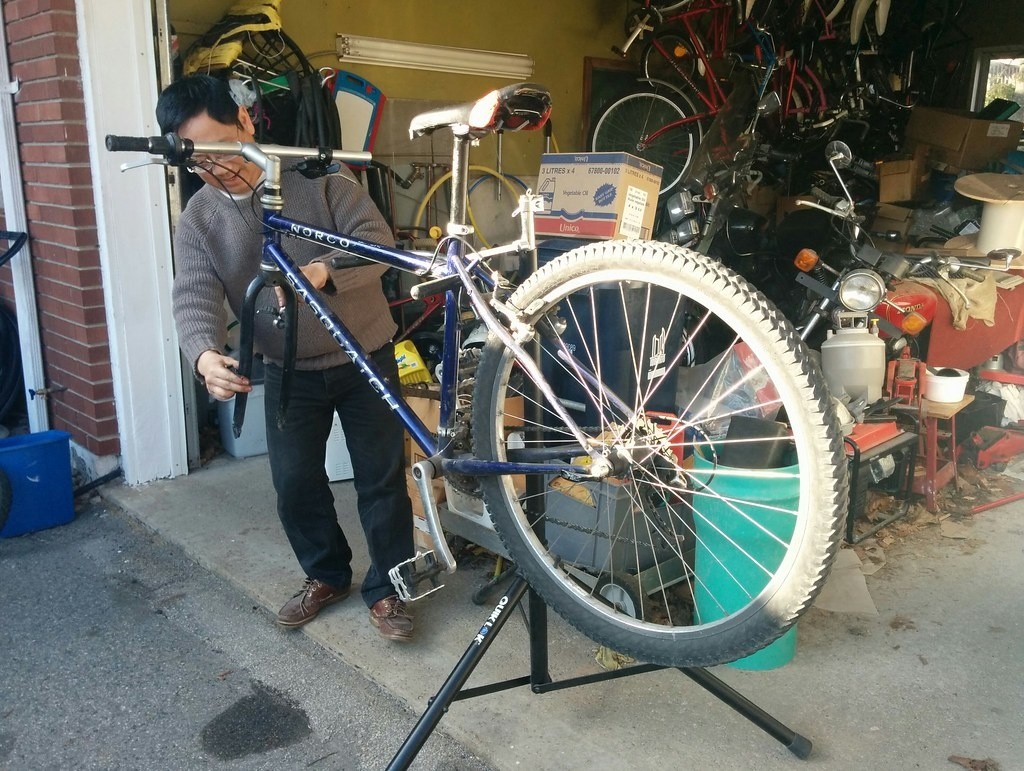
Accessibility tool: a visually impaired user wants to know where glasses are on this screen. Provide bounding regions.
[184,123,242,173]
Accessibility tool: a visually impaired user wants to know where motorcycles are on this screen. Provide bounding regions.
[780,140,1024,437]
[662,90,874,370]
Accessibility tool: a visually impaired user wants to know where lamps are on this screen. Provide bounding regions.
[336,33,535,81]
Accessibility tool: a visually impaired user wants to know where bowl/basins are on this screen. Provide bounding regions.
[922,367,970,403]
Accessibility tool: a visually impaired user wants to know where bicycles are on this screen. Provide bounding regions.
[103,81,849,669]
[583,0,805,201]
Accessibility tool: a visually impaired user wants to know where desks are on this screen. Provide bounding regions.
[892,394,977,512]
[775,412,920,548]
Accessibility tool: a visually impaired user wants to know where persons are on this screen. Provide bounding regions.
[153,75,417,645]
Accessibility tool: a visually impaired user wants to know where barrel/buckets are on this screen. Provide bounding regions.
[692,437,799,671]
[0,430,76,538]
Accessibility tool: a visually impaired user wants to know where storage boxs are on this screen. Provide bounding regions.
[536,454,694,575]
[906,107,1022,178]
[397,395,528,553]
[878,161,933,207]
[859,203,919,252]
[532,149,666,245]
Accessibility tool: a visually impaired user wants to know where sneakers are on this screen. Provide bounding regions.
[372,593,413,641]
[277,579,350,627]
